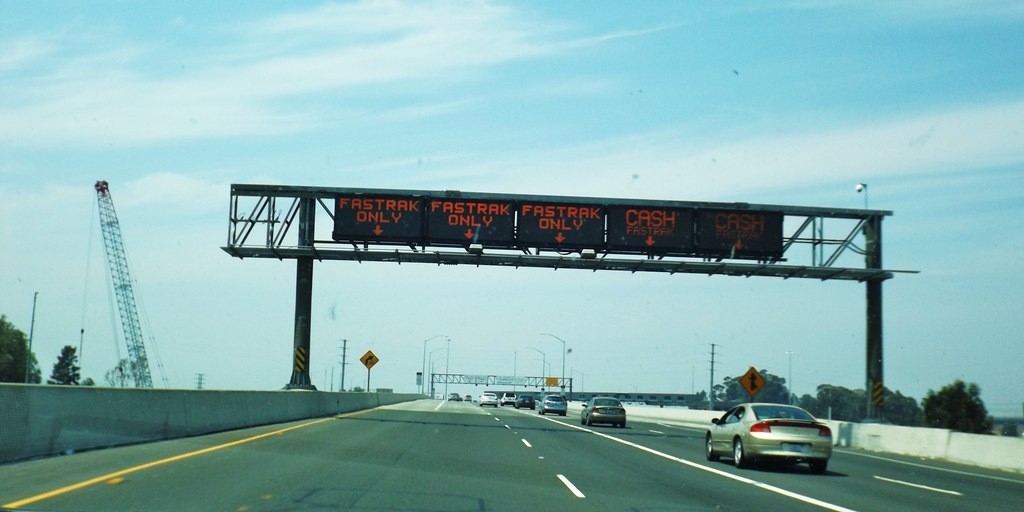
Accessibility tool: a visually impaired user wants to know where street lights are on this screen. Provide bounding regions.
[25,292,39,381]
[539,332,565,385]
[787,350,792,392]
[421,335,449,395]
[524,346,545,388]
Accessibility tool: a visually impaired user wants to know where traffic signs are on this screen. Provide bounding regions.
[516,202,606,252]
[606,205,695,253]
[332,196,425,243]
[426,197,516,247]
[695,208,783,258]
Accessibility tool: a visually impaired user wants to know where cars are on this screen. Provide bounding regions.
[561,395,568,407]
[500,393,516,407]
[515,395,535,410]
[448,393,460,402]
[705,403,832,473]
[478,393,498,408]
[537,395,567,416]
[464,395,472,402]
[580,397,626,428]
